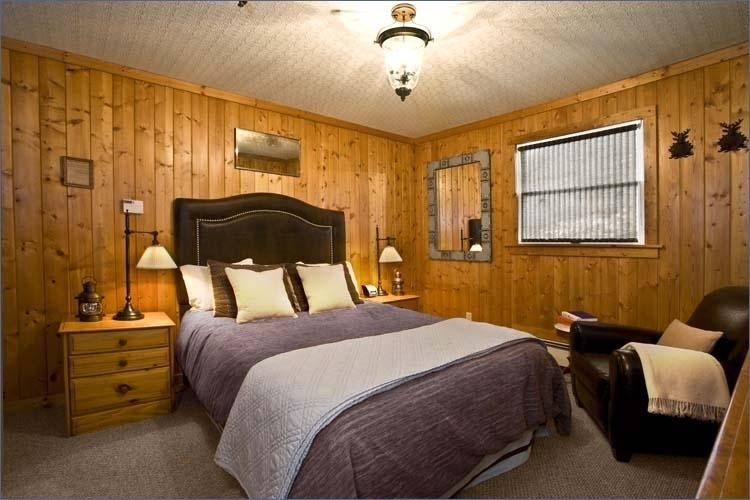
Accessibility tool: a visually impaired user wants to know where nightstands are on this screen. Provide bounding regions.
[359,292,420,312]
[57,312,176,438]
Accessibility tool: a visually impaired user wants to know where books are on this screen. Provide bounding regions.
[561,310,598,322]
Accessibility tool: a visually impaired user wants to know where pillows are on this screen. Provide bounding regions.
[296,264,357,315]
[207,258,301,317]
[655,318,723,354]
[285,261,364,312]
[223,266,298,324]
[179,258,253,312]
[294,261,361,300]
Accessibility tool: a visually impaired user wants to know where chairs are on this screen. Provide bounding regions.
[570,285,750,463]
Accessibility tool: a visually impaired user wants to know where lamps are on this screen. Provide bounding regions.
[376,224,403,296]
[461,228,482,252]
[113,209,178,320]
[375,4,435,101]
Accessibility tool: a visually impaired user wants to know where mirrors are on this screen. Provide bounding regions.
[427,150,491,261]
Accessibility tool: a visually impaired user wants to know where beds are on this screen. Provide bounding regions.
[175,192,572,500]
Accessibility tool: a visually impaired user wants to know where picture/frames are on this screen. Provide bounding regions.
[63,156,94,190]
[234,127,301,177]
